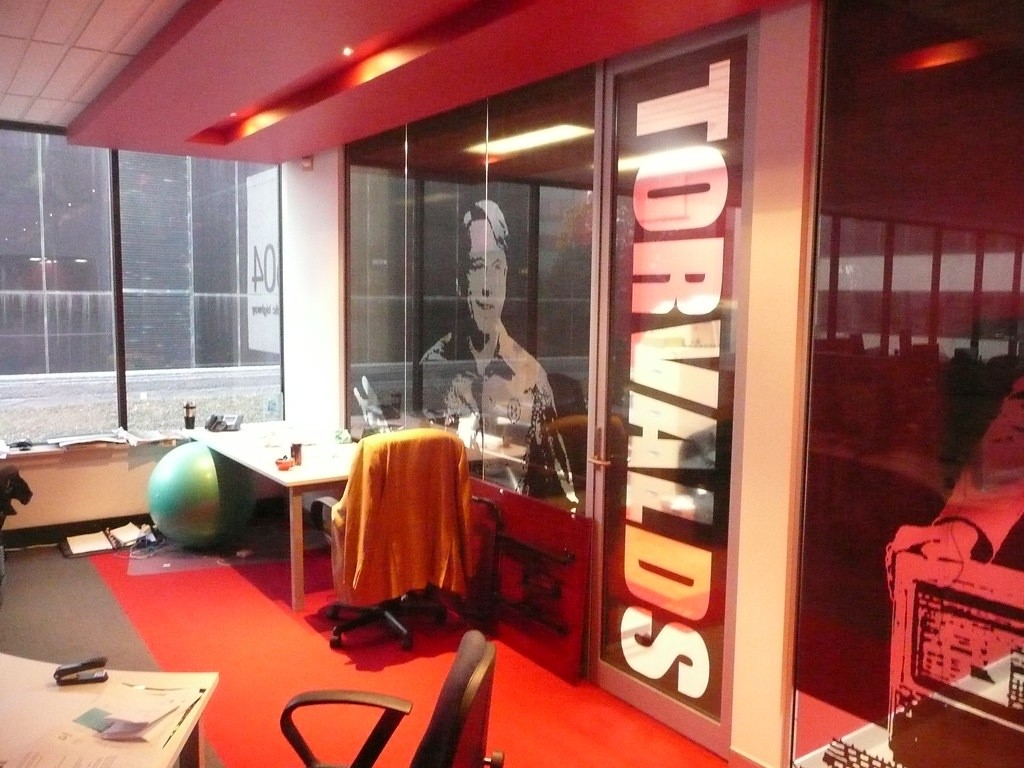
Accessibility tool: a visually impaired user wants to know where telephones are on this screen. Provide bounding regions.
[204,413,243,432]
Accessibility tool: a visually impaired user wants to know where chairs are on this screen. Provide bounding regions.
[280,630,504,768]
[310,428,480,652]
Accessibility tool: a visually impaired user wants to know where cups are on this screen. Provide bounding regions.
[184,400,196,429]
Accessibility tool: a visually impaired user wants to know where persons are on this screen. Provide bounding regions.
[352,200,580,506]
[885,381,1024,607]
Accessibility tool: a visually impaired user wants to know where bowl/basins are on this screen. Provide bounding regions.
[276,459,291,471]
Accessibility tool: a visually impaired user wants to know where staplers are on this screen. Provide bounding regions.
[53,655,109,686]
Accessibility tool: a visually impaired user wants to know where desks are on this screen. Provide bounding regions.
[0,652,220,768]
[182,421,358,611]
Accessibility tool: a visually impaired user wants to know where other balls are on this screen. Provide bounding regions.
[146,442,258,546]
